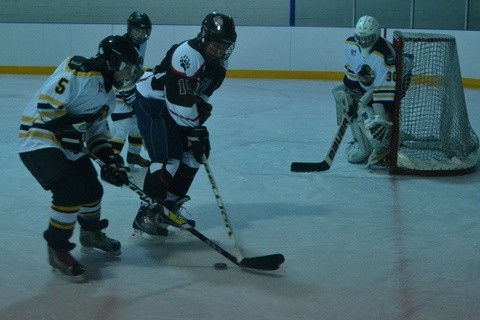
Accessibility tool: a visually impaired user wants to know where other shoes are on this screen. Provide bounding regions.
[127,152,150,171]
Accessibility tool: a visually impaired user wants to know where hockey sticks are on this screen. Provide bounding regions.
[81,145,281,271]
[202,154,285,264]
[291,98,358,172]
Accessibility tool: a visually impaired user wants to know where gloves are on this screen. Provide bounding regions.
[55,122,88,154]
[196,101,212,124]
[96,148,129,187]
[119,88,136,108]
[186,126,210,164]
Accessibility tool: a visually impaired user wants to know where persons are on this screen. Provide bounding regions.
[332,15,415,171]
[104,10,151,176]
[133,11,236,242]
[18,35,142,280]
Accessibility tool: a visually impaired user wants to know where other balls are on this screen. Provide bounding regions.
[214,263,227,269]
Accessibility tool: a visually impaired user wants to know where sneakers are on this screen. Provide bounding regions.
[163,195,195,232]
[44,229,87,282]
[133,198,168,240]
[77,215,121,257]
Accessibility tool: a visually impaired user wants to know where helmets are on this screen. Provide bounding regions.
[200,12,237,63]
[354,16,380,55]
[127,11,152,45]
[97,35,140,72]
